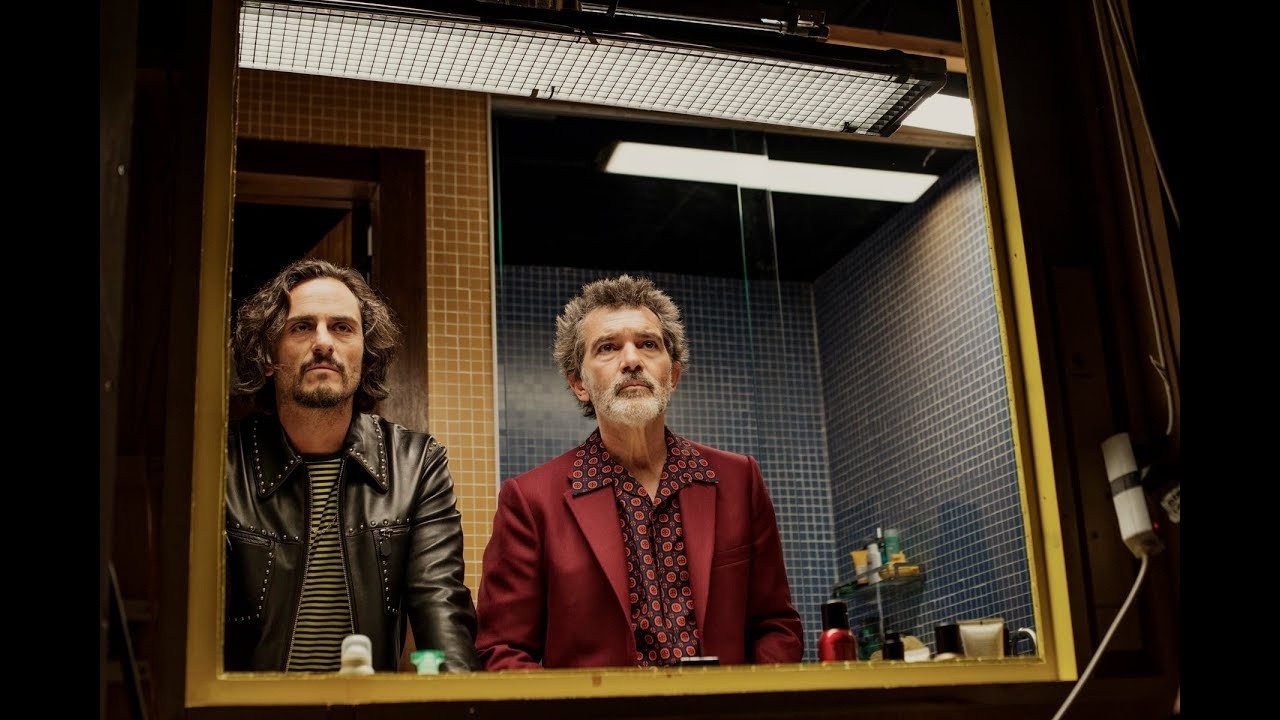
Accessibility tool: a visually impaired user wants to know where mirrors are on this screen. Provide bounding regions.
[187,1,1080,706]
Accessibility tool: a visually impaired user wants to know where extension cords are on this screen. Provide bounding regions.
[1102,434,1163,560]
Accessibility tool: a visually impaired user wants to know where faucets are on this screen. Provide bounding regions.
[1009,627,1038,656]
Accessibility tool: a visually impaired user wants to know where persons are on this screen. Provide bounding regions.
[220,256,478,676]
[472,274,807,673]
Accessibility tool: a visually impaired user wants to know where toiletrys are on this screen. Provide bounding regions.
[935,617,1011,661]
[337,634,377,674]
[859,628,883,661]
[851,528,900,589]
[818,599,858,661]
[882,630,905,660]
[410,648,447,674]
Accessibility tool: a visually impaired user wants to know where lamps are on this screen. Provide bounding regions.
[239,0,949,138]
